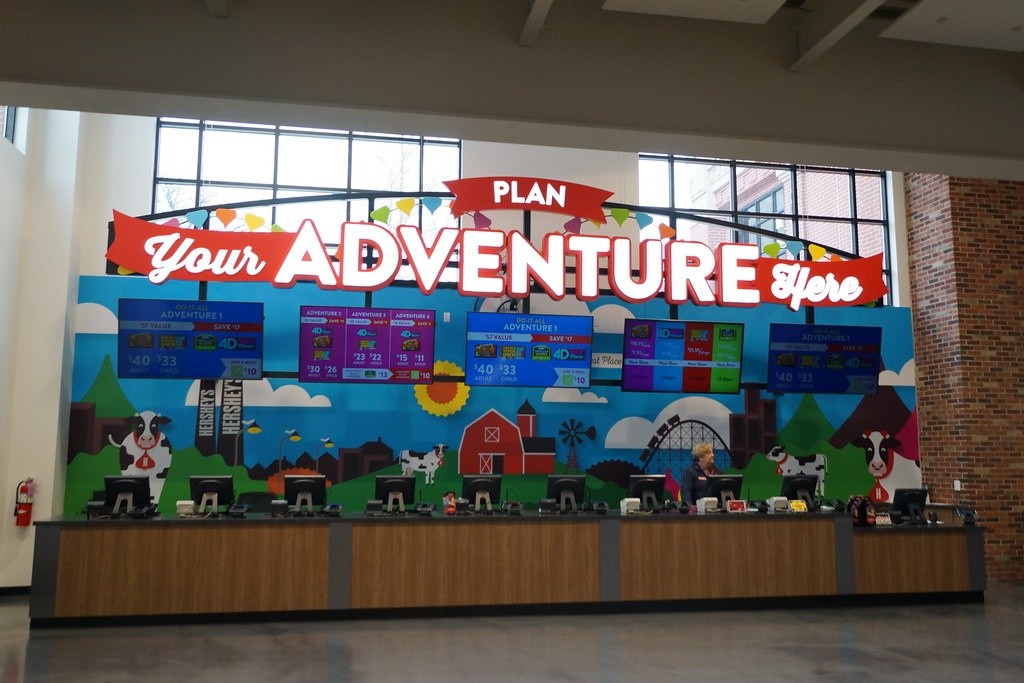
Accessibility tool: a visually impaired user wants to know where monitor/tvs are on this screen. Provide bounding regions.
[706,474,743,508]
[627,474,666,509]
[189,476,235,518]
[375,475,416,513]
[546,475,587,514]
[462,474,503,513]
[101,475,151,515]
[778,474,818,510]
[284,474,327,514]
[893,488,928,523]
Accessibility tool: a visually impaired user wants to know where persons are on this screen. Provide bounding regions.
[682,442,722,511]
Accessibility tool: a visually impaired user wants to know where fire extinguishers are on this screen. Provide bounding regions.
[13,477,35,527]
